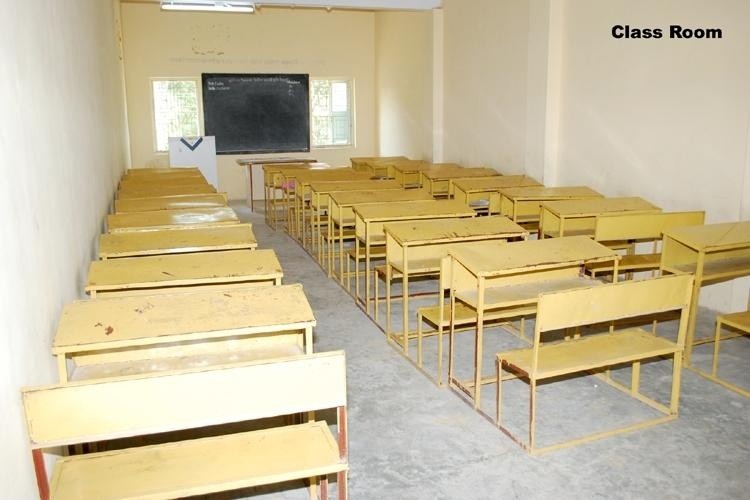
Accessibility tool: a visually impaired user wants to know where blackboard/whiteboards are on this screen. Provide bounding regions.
[201,73,310,155]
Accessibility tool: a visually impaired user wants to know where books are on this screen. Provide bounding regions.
[470,199,490,207]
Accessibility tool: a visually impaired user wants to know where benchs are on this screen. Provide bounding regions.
[23,351,350,500]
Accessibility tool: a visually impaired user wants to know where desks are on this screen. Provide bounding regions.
[48,155,749,456]
